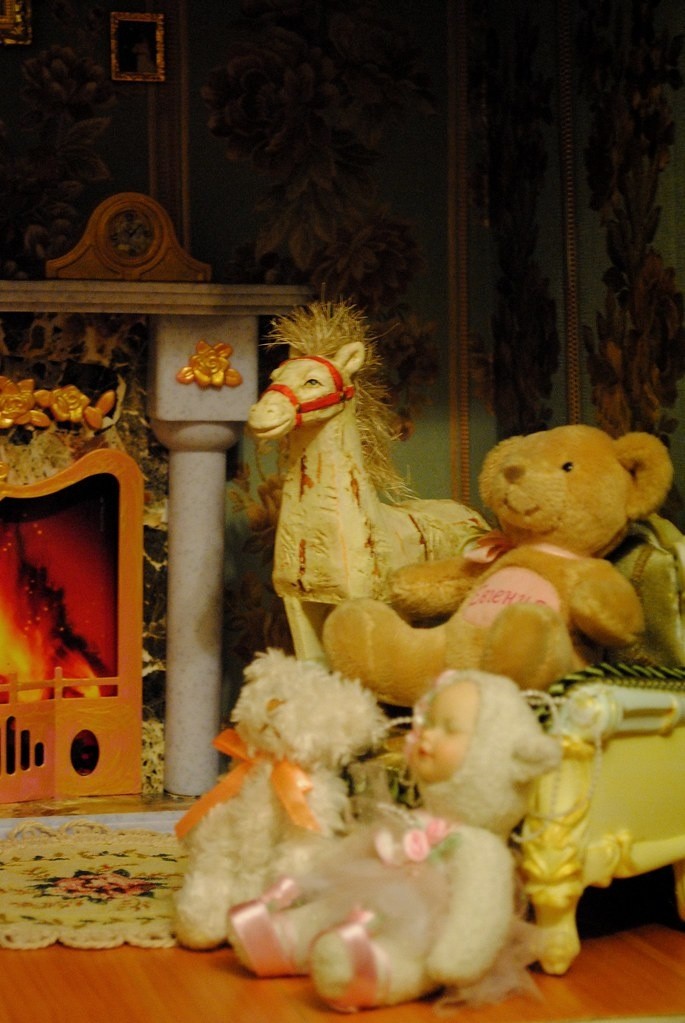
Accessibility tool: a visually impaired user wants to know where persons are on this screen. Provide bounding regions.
[226,669,565,1013]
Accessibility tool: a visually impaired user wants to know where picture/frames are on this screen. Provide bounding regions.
[1,0,35,46]
[109,12,166,81]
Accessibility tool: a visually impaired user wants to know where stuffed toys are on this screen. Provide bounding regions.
[320,425,674,709]
[172,653,390,949]
